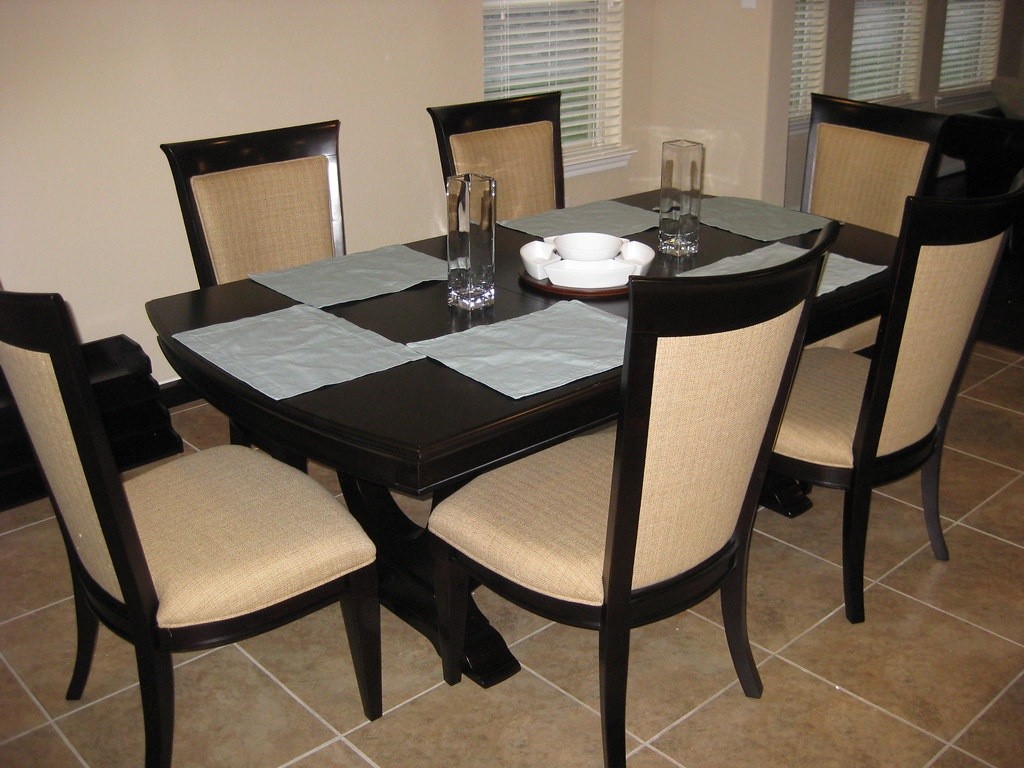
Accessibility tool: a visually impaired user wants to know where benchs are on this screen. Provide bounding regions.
[0,335,183,513]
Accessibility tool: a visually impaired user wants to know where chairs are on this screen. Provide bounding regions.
[0,290,382,768]
[799,92,954,493]
[160,121,346,474]
[769,168,1024,626]
[423,221,843,768]
[427,90,567,226]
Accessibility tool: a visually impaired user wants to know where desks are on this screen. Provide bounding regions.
[141,187,898,689]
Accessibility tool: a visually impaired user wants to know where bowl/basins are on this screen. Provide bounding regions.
[519,233,655,288]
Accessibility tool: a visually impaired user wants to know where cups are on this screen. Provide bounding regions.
[445,172,496,310]
[658,140,704,256]
